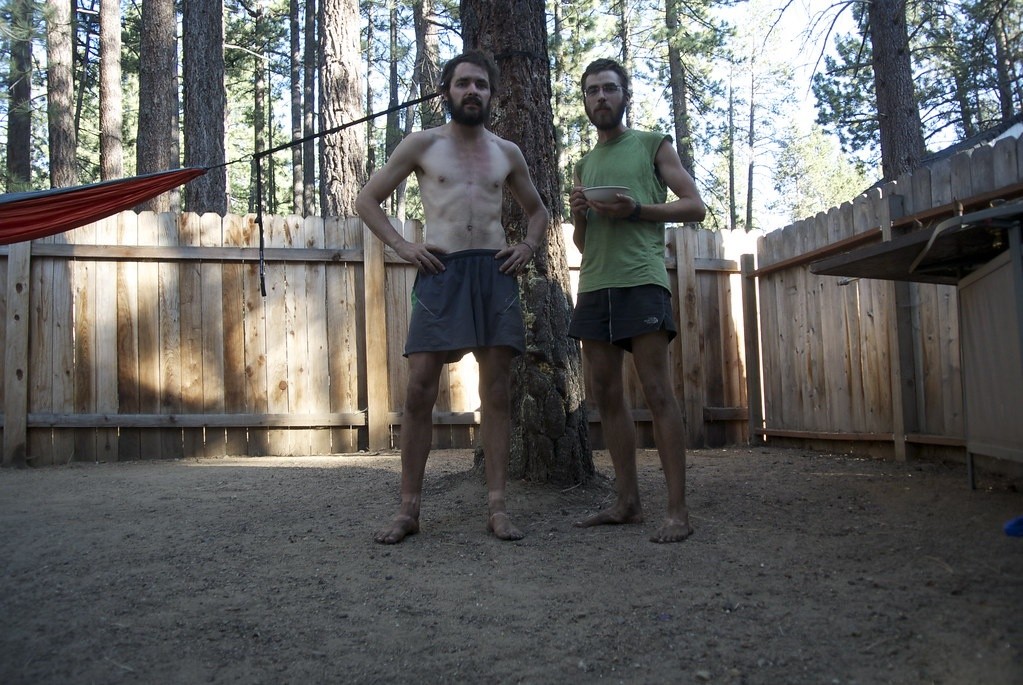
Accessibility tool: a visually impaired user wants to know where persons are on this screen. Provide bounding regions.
[569,59,706,545]
[355,47,549,544]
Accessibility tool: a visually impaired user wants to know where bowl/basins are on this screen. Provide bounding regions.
[581,186,630,202]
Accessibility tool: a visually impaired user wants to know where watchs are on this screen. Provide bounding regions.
[623,202,642,224]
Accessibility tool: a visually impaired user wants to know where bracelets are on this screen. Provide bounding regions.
[521,243,534,254]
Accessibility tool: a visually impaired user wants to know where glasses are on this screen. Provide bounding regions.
[582,83,628,98]
